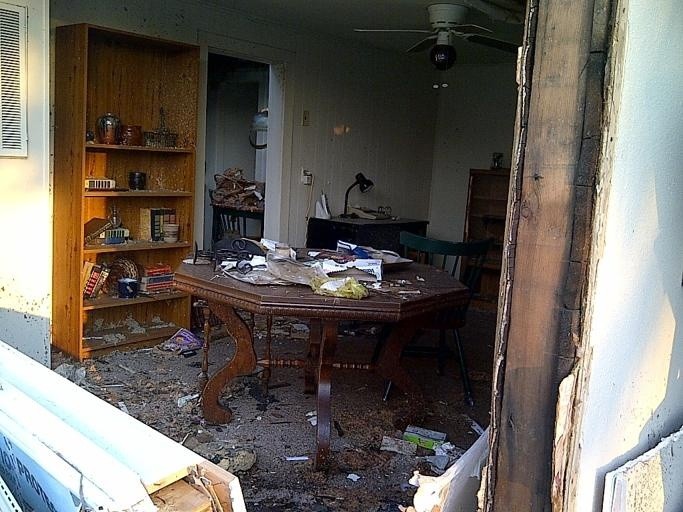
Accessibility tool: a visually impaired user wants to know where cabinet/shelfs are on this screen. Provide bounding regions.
[50,21,199,366]
[459,166,512,315]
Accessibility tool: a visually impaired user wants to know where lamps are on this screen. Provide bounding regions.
[429,40,460,75]
[340,172,377,220]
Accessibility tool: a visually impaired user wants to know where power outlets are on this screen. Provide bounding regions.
[301,166,313,187]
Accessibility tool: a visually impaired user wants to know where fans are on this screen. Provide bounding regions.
[355,1,526,64]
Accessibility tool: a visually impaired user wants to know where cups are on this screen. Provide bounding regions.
[162,222,179,244]
[127,171,147,190]
[117,277,138,298]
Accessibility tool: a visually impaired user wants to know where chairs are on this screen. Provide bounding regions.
[374,232,484,411]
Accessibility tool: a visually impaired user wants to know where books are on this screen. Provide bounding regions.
[152,209,175,241]
[82,258,112,300]
[139,262,175,294]
[83,173,130,192]
[83,216,129,244]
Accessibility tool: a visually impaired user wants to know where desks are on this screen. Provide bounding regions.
[172,246,475,473]
[209,189,263,246]
[304,217,428,265]
